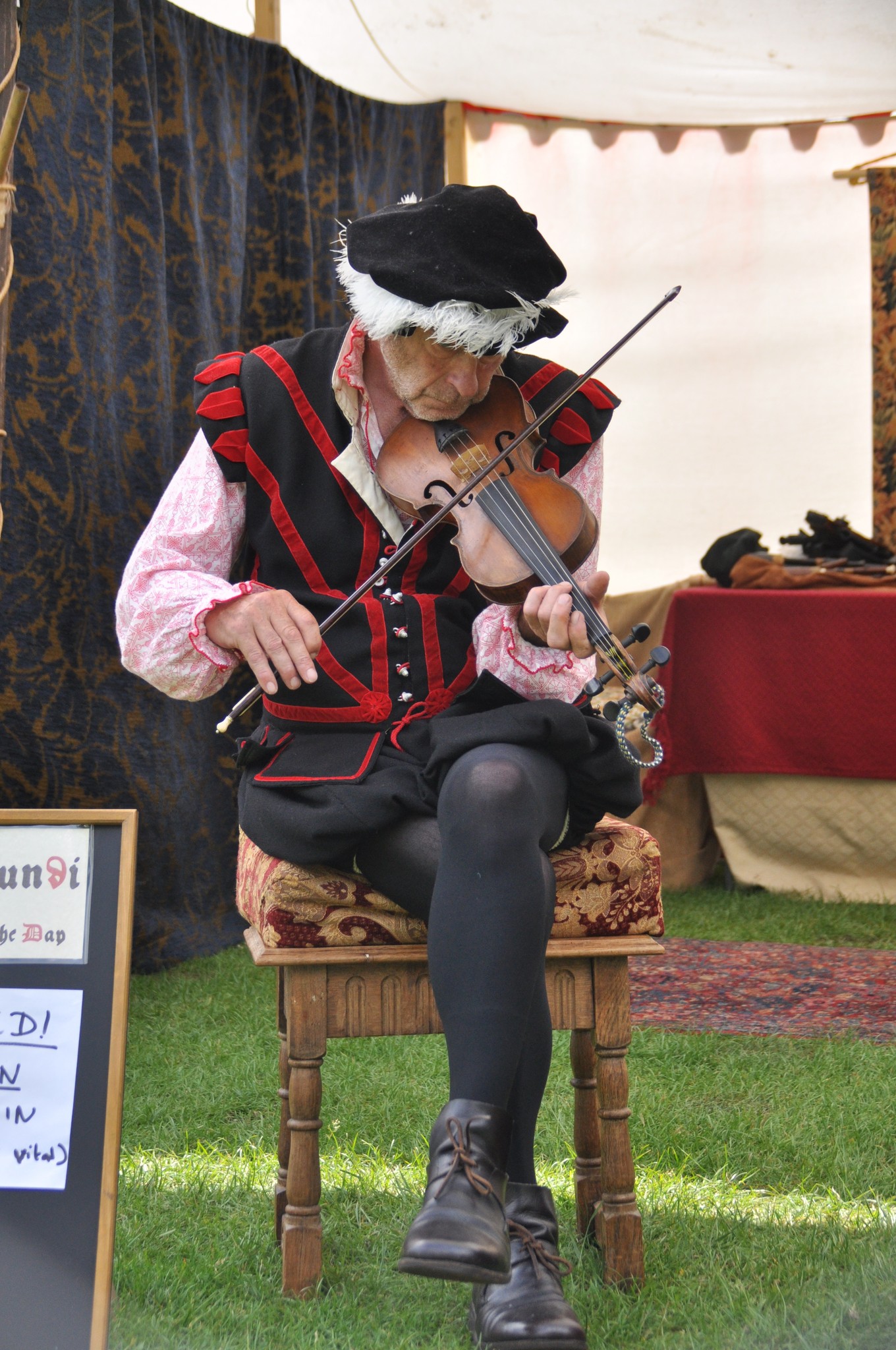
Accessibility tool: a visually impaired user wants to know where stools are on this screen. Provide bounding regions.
[235,813,668,1301]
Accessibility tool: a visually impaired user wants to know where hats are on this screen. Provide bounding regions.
[335,182,570,355]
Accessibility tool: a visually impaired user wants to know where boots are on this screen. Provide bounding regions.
[396,1099,514,1284]
[468,1181,586,1350]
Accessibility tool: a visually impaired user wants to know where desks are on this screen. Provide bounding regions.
[643,583,895,905]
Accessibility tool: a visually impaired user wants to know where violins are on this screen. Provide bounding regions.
[375,374,672,722]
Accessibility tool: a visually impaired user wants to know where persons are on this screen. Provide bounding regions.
[115,189,626,1350]
